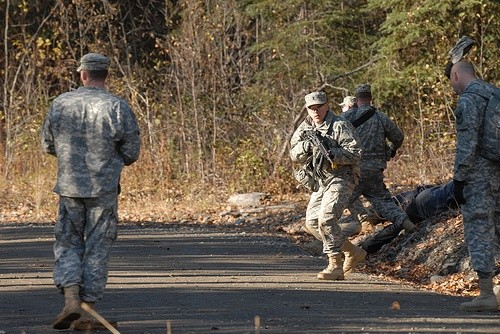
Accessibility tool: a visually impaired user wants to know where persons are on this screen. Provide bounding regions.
[43,53,142,332]
[290,91,366,279]
[445,60,500,312]
[359,180,457,254]
[338,84,420,235]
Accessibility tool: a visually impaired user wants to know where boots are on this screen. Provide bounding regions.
[74,300,95,331]
[53,285,82,330]
[340,240,367,274]
[316,256,344,280]
[459,278,497,312]
[472,286,500,311]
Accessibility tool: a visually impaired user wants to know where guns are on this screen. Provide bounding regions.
[308,129,339,170]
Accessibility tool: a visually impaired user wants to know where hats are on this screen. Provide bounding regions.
[448,36,475,64]
[77,53,110,73]
[338,96,356,106]
[305,91,326,108]
[354,84,371,93]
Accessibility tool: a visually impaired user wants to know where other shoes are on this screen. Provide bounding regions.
[402,218,418,233]
